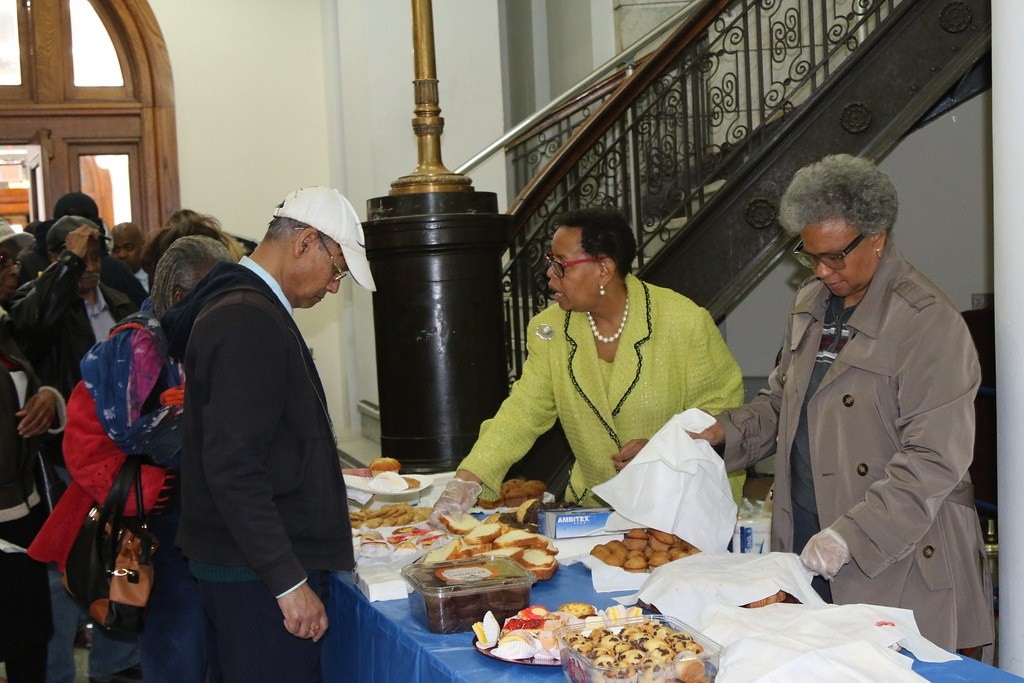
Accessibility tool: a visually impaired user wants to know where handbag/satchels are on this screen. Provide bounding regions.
[61,452,160,646]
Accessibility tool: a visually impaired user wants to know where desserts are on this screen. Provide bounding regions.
[591,527,701,573]
[472,603,643,660]
[352,526,453,558]
[477,478,548,510]
[484,499,583,535]
[564,625,714,683]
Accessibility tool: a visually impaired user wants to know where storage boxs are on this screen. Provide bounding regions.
[536,507,630,540]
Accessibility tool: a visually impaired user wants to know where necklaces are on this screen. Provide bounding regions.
[587,293,628,343]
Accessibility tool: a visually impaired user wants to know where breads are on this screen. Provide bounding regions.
[370,457,401,476]
[422,523,560,579]
[439,513,481,534]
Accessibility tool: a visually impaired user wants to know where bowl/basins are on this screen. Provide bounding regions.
[551,614,728,683]
[400,554,538,634]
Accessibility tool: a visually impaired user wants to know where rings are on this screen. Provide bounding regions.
[310,624,320,635]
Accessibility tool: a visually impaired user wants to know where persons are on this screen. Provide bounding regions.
[0,192,259,683]
[686,153,994,658]
[161,186,378,682]
[427,206,746,535]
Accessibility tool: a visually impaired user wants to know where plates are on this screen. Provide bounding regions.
[343,474,434,495]
[472,625,562,667]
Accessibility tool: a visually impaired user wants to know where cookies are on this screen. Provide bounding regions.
[348,502,434,529]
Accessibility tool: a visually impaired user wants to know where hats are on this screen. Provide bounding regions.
[0,216,36,255]
[273,186,376,293]
[45,214,111,252]
[54,191,106,234]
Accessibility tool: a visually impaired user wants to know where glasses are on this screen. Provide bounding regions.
[791,232,866,271]
[0,252,24,272]
[543,251,601,278]
[294,227,347,280]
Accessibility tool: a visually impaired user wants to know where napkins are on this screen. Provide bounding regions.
[342,472,409,505]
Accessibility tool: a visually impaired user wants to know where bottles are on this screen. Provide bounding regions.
[979,517,998,599]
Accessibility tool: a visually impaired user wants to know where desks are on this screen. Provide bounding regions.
[321,472,1024,683]
